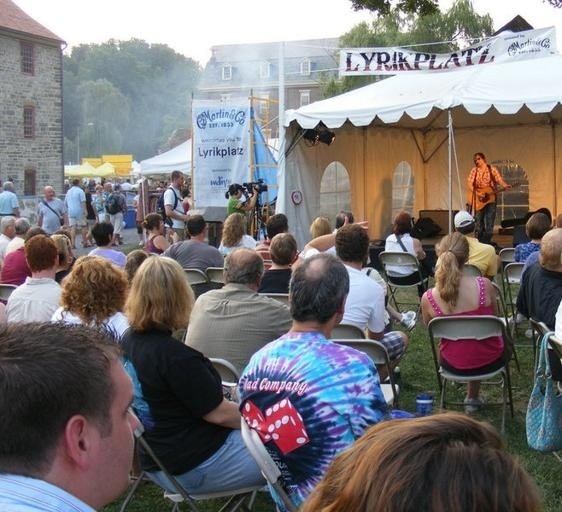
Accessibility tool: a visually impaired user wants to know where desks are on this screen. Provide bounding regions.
[419,210,457,237]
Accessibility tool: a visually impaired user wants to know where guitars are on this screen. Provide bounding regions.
[467,180,521,211]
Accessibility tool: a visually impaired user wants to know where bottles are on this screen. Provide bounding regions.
[415,393,434,415]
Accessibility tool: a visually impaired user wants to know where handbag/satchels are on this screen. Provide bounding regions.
[59,216,64,226]
[526,369,562,451]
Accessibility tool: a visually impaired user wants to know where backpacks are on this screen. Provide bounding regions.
[157,191,165,214]
[105,195,122,215]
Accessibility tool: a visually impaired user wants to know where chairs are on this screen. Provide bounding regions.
[0,283,19,305]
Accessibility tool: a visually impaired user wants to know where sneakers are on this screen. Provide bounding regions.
[464,398,485,414]
[401,310,416,332]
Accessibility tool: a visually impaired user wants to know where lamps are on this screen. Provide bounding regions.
[286,129,336,156]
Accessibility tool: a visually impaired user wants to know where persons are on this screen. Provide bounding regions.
[50,233,73,284]
[516,227,562,385]
[155,181,167,191]
[217,212,257,257]
[141,212,170,255]
[63,179,91,249]
[384,212,434,304]
[104,183,113,193]
[164,170,191,244]
[105,185,127,246]
[334,210,354,233]
[513,213,552,262]
[81,185,100,244]
[6,234,66,326]
[0,317,141,512]
[3,217,32,261]
[0,215,18,263]
[184,248,294,384]
[36,185,68,235]
[133,190,150,246]
[256,213,300,272]
[361,259,417,332]
[310,217,332,239]
[0,227,48,305]
[304,216,408,383]
[421,232,513,413]
[0,181,20,222]
[51,256,132,340]
[124,250,148,281]
[92,185,107,224]
[297,408,546,512]
[259,233,298,293]
[455,210,502,282]
[120,257,268,496]
[121,179,133,190]
[88,220,127,269]
[468,152,513,242]
[161,214,224,270]
[236,253,417,512]
[225,183,260,234]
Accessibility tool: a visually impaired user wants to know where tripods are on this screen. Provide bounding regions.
[248,201,267,241]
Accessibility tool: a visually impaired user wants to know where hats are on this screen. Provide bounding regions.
[395,211,412,224]
[453,210,475,228]
